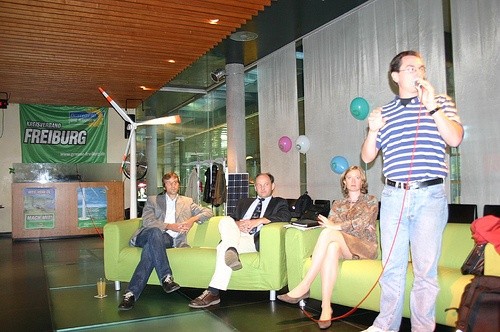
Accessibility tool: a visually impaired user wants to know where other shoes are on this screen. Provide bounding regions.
[160,275,180,293]
[117,291,136,310]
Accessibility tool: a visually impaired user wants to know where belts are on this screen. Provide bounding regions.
[384,177,444,190]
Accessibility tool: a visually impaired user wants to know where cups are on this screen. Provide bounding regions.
[97,278,105,297]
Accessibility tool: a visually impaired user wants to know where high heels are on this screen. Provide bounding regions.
[277,288,310,304]
[318,308,332,328]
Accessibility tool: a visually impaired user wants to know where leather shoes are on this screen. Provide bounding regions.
[188,289,221,308]
[224,246,243,272]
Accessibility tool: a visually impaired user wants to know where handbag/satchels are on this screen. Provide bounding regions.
[460,244,485,276]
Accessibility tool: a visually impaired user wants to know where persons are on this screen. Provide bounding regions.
[188,172,291,308]
[277,165,378,329]
[359,51,465,332]
[118,172,215,310]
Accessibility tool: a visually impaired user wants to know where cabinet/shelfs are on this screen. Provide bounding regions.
[11,181,124,240]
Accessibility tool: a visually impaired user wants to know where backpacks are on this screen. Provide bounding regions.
[445,274,500,331]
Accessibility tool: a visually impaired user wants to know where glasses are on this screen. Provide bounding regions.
[399,65,426,73]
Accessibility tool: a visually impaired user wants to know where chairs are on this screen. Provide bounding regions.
[284,200,500,225]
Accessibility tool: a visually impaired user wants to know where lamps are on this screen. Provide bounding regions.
[209,68,226,84]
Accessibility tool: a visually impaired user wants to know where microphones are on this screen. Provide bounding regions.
[414,80,423,89]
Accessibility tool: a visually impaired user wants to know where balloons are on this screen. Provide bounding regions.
[350,97,369,120]
[295,135,311,153]
[279,136,292,152]
[330,156,349,174]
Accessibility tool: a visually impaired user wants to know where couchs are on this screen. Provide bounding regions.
[285,224,500,328]
[103,218,291,301]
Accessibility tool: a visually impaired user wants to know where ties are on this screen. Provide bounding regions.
[247,198,265,235]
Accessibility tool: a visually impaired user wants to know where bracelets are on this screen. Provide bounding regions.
[429,106,442,115]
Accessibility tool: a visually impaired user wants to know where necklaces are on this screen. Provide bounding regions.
[348,201,357,203]
